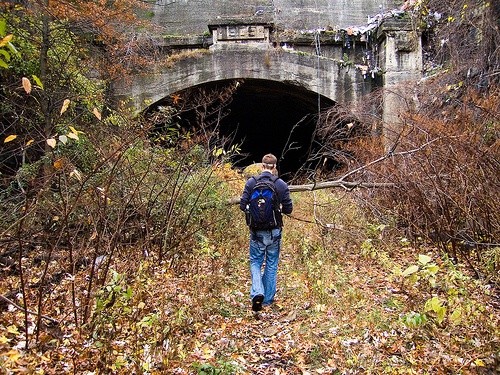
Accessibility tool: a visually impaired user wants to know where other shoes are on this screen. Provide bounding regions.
[252,294,264,311]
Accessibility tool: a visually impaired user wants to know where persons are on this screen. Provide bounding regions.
[239,154,293,312]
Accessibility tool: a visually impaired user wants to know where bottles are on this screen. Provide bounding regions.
[244,205,251,225]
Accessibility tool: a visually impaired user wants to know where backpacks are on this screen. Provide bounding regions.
[245,175,281,240]
